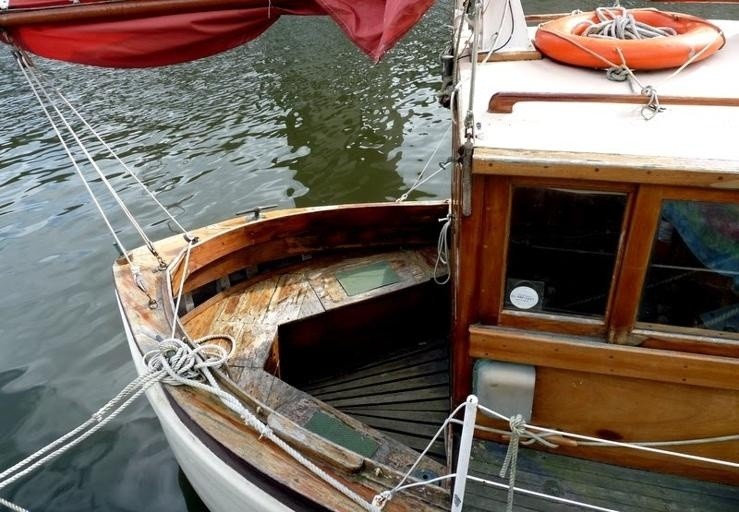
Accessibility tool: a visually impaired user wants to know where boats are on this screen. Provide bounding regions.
[79,0,739,512]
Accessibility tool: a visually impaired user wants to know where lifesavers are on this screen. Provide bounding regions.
[533,8,726,69]
